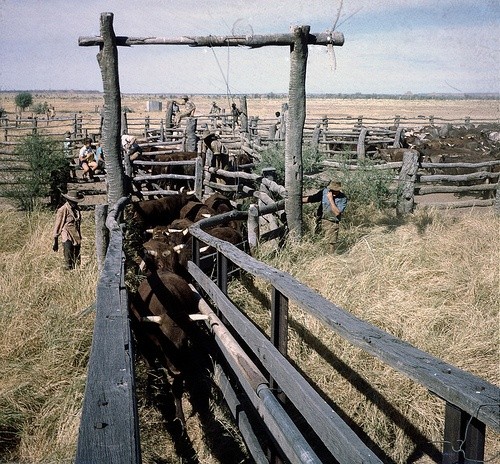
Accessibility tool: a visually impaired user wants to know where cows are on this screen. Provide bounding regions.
[135,145,198,190]
[122,188,243,418]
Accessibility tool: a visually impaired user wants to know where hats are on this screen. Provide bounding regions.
[181,95,188,99]
[200,129,215,139]
[211,101,216,105]
[61,189,86,203]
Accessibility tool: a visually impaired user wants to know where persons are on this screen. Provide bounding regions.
[303,179,347,253]
[229,103,240,133]
[274,112,281,139]
[210,101,221,129]
[53,189,85,271]
[46,107,56,126]
[120,134,142,178]
[171,96,196,129]
[63,130,106,181]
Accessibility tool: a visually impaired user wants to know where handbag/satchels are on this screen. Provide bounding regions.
[129,151,141,160]
[127,142,139,156]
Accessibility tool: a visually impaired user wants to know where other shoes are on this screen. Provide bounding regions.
[101,168,104,173]
